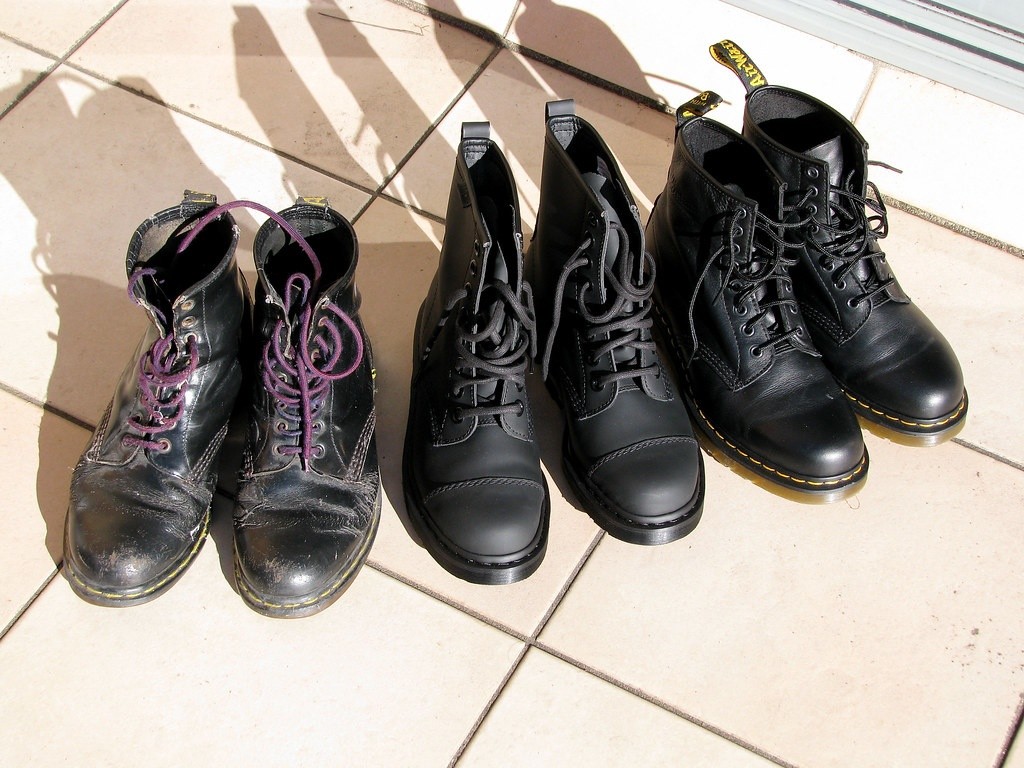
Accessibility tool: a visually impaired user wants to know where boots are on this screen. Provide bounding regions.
[644,91,870,508]
[232,195,381,619]
[522,98,706,546]
[709,38,969,449]
[66,189,256,607]
[401,123,550,585]
